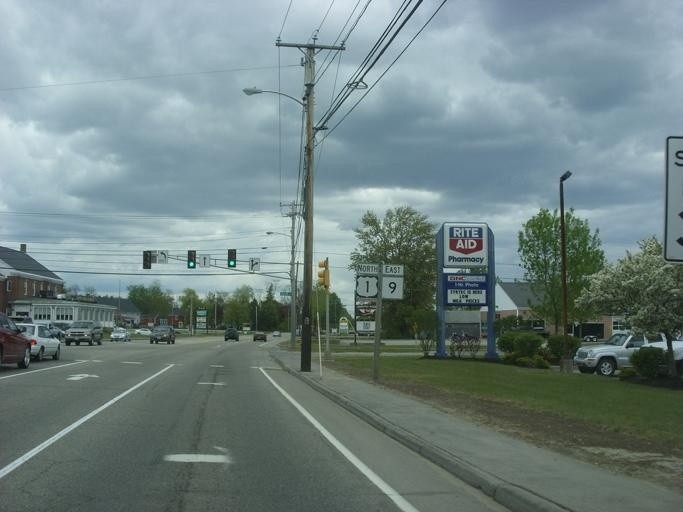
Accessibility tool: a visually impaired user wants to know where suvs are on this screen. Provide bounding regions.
[224,327,240,342]
[65,319,104,346]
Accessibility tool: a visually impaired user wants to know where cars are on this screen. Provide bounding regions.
[253,331,267,342]
[273,330,282,337]
[135,327,152,335]
[110,329,132,343]
[1,310,33,369]
[13,323,62,360]
[149,325,176,344]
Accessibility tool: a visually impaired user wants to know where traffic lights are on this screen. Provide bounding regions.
[316,256,330,288]
[143,251,152,270]
[188,250,197,269]
[228,249,237,268]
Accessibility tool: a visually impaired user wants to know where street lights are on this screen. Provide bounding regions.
[261,244,291,250]
[267,229,295,346]
[558,172,570,335]
[242,42,348,372]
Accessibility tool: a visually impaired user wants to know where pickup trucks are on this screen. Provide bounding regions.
[572,329,683,377]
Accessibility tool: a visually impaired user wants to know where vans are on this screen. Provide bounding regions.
[35,319,63,340]
